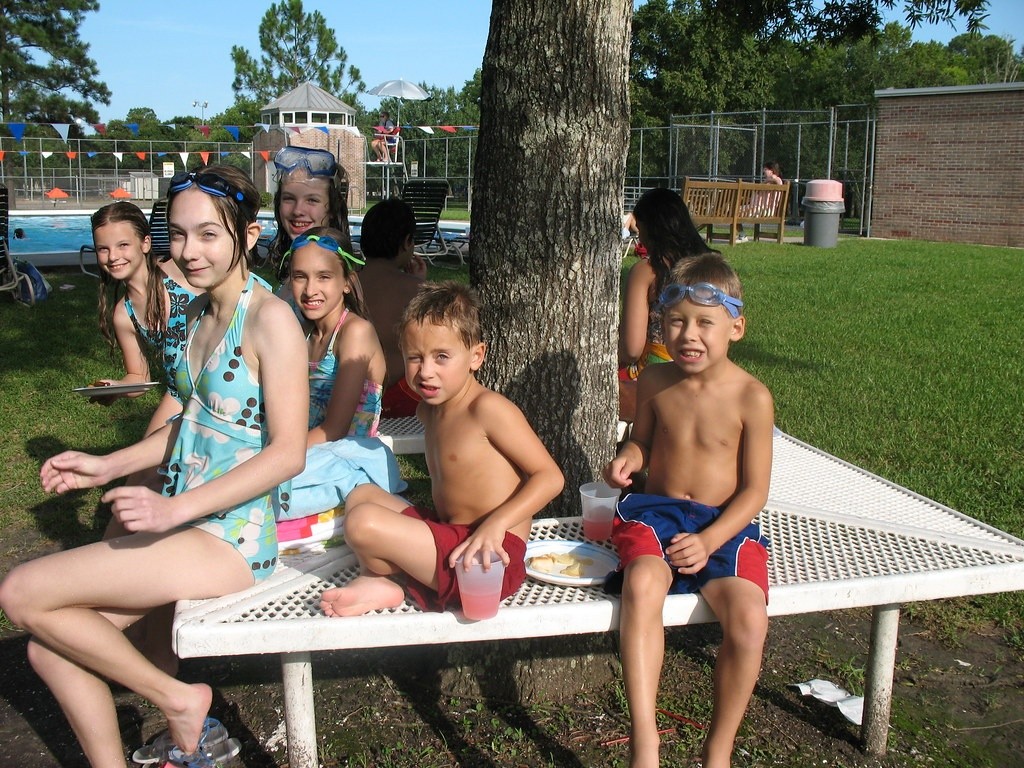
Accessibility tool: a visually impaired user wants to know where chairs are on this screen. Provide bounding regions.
[374,126,398,161]
[80,196,169,278]
[351,178,471,269]
[249,228,281,266]
[0,182,36,309]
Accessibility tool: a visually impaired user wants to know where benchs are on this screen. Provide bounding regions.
[680,177,790,245]
[167,394,1024,768]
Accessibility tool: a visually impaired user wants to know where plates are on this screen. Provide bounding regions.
[72,382,162,396]
[523,541,621,587]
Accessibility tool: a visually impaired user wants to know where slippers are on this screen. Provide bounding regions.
[132,716,242,768]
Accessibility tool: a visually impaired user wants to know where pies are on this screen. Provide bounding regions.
[530,553,594,576]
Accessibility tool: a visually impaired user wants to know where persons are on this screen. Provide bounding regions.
[617,210,640,241]
[616,187,715,383]
[87,204,274,541]
[599,250,775,768]
[124,235,388,676]
[253,142,350,328]
[317,281,565,620]
[733,160,782,244]
[354,197,429,419]
[0,165,310,768]
[371,111,395,162]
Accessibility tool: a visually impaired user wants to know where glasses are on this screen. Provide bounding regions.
[169,172,231,197]
[658,281,725,307]
[290,235,338,251]
[273,145,337,176]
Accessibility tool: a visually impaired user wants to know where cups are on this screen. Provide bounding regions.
[455,552,505,621]
[579,482,622,541]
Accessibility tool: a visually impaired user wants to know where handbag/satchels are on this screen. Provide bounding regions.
[13,256,52,303]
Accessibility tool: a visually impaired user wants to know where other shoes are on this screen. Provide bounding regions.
[735,236,748,243]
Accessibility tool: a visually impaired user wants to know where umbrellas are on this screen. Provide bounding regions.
[369,75,430,140]
[109,187,131,202]
[45,187,68,206]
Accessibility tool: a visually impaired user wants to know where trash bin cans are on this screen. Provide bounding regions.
[802,178,845,247]
